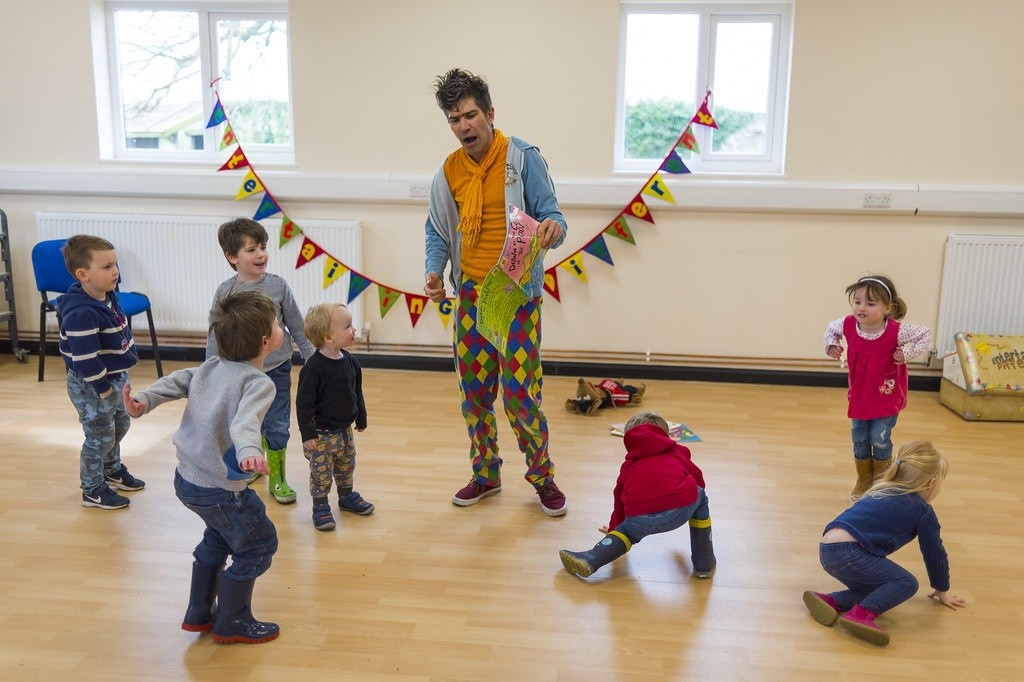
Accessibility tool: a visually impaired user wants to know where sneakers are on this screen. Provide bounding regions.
[82,484,130,510]
[104,463,145,491]
[338,491,374,515]
[841,605,889,647]
[312,504,336,530]
[453,479,501,506]
[534,482,567,516]
[803,591,841,627]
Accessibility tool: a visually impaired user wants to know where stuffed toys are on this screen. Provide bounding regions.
[566,378,646,416]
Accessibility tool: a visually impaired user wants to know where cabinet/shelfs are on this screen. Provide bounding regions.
[0,207,32,364]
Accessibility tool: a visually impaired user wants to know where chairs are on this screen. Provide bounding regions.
[32,238,163,384]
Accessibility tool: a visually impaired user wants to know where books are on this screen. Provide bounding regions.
[476,203,549,357]
[610,411,680,438]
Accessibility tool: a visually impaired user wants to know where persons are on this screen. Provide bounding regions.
[422,68,568,517]
[123,290,284,643]
[824,275,931,502]
[296,301,374,530]
[559,408,717,579]
[205,219,316,505]
[803,438,966,645]
[56,234,146,509]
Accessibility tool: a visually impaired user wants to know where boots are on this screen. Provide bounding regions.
[851,457,872,499]
[181,561,227,632]
[559,530,631,578]
[690,518,716,578]
[246,435,267,484]
[212,571,280,644]
[266,449,297,503]
[872,457,893,481]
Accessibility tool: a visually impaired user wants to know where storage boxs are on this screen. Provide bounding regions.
[936,331,1024,423]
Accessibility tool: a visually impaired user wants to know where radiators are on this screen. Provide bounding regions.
[32,211,363,340]
[936,232,1024,358]
